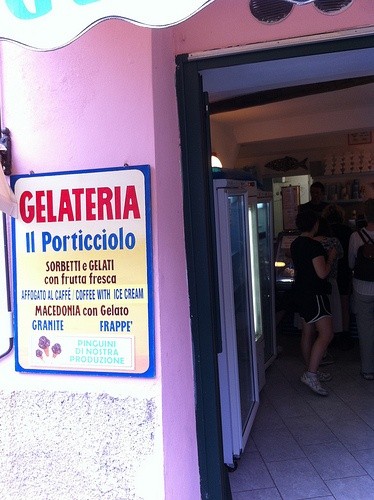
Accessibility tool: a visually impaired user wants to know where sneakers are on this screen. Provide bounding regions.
[300,372,328,396]
[319,352,335,364]
[316,369,332,381]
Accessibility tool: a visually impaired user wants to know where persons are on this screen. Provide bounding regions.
[321,203,354,351]
[312,215,342,364]
[348,198,374,379]
[295,181,329,224]
[290,208,336,397]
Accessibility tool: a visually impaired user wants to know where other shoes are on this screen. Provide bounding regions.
[362,373,374,379]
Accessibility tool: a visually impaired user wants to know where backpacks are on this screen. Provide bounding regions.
[353,229,374,283]
